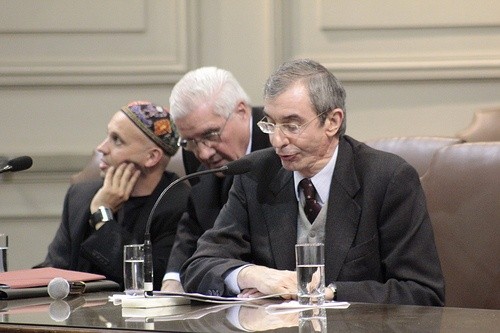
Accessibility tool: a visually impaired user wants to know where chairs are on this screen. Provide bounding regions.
[362,110,500,310]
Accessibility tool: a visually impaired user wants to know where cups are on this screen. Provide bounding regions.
[296,309,327,333]
[123,243,144,297]
[295,243,325,305]
[124,317,146,330]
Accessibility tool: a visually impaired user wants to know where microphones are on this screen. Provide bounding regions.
[120,159,256,310]
[47,278,86,299]
[48,295,86,322]
[0,156,32,174]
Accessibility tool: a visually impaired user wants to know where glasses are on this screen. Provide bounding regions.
[178,110,231,151]
[257,109,330,137]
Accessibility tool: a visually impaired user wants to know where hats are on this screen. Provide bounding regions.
[121,101,179,156]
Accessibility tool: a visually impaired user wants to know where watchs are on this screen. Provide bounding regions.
[89,205,113,226]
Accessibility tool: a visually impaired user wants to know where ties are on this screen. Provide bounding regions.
[300,178,322,223]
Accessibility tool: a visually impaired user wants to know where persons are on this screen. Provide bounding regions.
[33,102,190,292]
[159,67,272,294]
[65,300,323,333]
[179,60,445,308]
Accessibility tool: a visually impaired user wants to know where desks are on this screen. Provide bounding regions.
[0,294,500,333]
